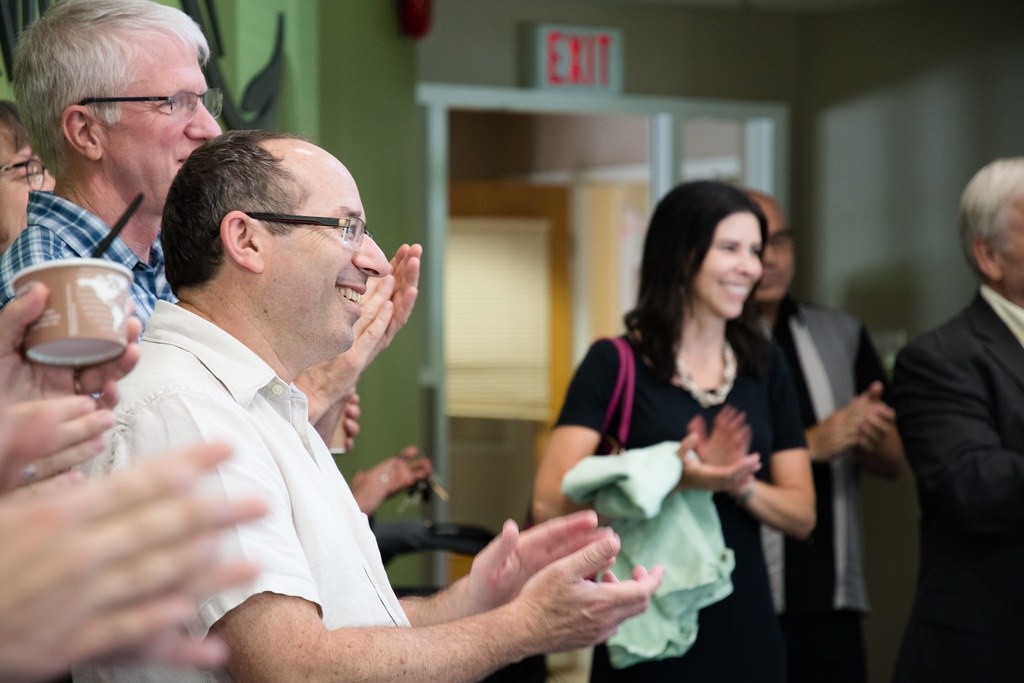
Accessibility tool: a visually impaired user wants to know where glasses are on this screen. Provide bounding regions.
[243,210,374,248]
[3,160,53,190]
[81,89,223,123]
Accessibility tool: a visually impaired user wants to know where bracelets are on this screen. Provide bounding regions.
[743,475,756,499]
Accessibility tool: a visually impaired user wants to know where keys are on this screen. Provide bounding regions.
[396,475,449,516]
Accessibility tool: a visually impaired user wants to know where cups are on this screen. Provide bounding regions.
[8,258,134,369]
[317,408,347,456]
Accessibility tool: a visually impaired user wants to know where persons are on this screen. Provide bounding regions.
[531,182,817,683]
[0,100,59,258]
[0,0,425,451]
[314,382,431,518]
[0,442,272,683]
[891,159,1024,683]
[743,190,900,683]
[65,128,666,683]
[0,279,140,492]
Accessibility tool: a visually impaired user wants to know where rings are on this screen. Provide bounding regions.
[73,378,82,393]
[17,463,36,482]
[379,474,388,483]
